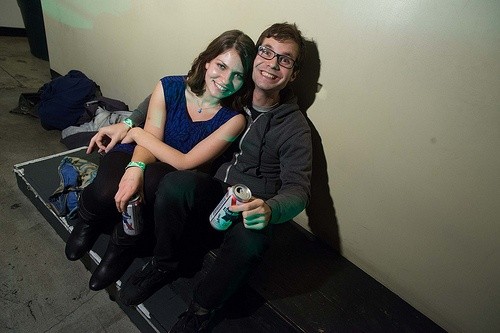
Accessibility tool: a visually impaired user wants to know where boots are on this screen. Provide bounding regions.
[64,192,107,261]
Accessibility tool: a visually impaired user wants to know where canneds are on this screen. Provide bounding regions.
[209,184,251,230]
[122,194,145,236]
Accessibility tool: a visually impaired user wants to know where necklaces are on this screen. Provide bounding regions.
[196,96,220,113]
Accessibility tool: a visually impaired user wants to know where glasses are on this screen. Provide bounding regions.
[257,44,298,69]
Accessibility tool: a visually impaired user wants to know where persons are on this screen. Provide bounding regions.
[65,30,256,291]
[86,21,312,333]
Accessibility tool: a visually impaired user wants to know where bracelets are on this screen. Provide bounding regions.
[125,161,146,172]
[122,117,135,128]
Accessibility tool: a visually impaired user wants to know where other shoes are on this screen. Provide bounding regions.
[168,302,231,333]
[120,253,188,306]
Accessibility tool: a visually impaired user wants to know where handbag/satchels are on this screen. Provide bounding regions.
[98,95,129,112]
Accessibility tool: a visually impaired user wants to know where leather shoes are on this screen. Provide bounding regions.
[88,220,141,291]
